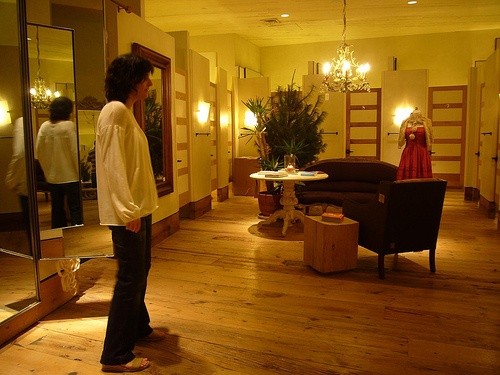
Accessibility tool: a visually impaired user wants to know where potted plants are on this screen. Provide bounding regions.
[240,69,328,216]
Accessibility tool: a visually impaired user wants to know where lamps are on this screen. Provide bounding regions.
[30,25,60,110]
[323,0,371,94]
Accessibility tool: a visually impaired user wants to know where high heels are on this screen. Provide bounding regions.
[134,329,165,343]
[101,357,150,371]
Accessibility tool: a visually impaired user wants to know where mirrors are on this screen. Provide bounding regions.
[0,0,115,325]
[27,23,84,231]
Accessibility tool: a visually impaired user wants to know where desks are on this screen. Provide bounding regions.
[303,216,358,274]
[249,171,328,236]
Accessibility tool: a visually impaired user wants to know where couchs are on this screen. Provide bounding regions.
[273,159,399,203]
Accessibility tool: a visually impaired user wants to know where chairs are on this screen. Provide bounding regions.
[342,178,447,279]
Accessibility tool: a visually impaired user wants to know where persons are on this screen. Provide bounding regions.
[7,115,32,257]
[89,54,166,372]
[34,95,85,230]
[398,107,435,183]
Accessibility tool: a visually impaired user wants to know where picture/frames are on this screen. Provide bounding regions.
[131,42,174,198]
